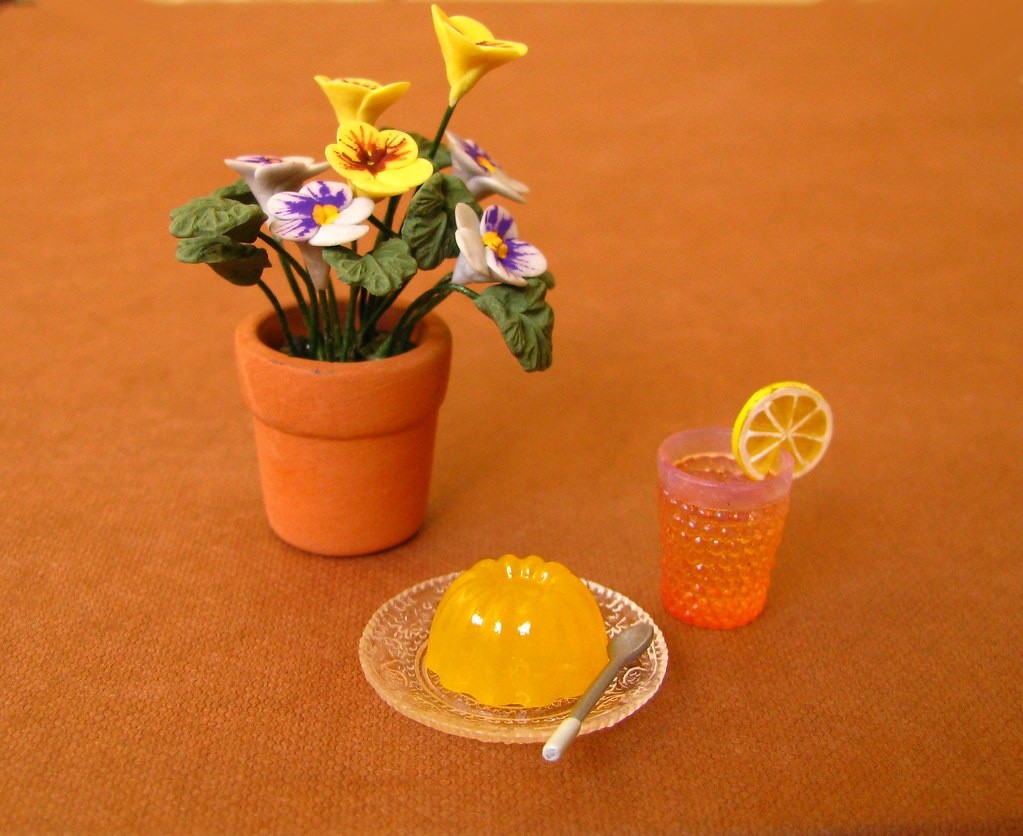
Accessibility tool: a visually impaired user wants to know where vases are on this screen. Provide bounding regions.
[236,298,453,555]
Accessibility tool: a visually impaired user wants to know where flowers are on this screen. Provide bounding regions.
[167,3,553,375]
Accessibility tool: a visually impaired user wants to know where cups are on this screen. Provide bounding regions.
[657,428,794,633]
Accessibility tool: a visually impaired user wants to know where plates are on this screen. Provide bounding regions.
[360,569,669,745]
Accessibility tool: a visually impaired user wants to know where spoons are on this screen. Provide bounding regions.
[541,623,656,761]
